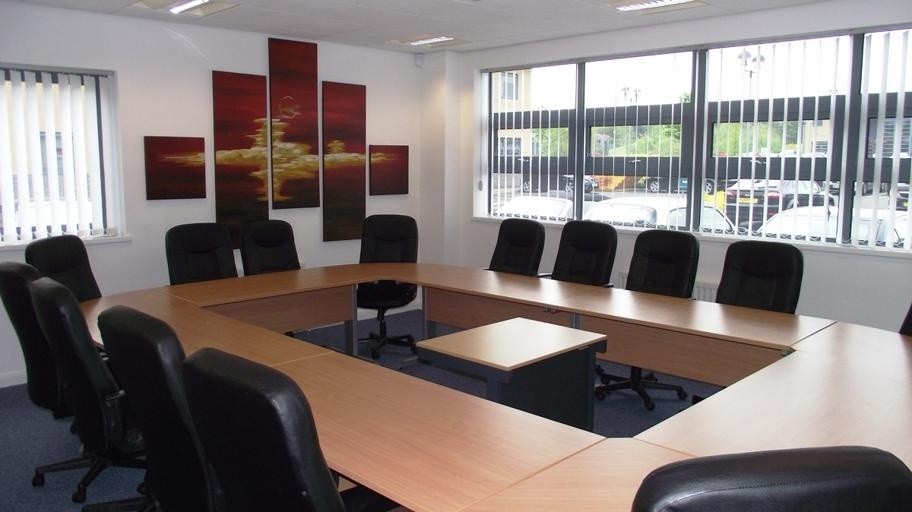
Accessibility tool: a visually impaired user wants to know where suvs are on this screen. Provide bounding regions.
[525,168,597,193]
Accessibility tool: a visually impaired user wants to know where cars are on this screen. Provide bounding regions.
[888,182,910,208]
[499,196,578,220]
[643,172,721,194]
[1,198,93,241]
[584,199,744,236]
[823,177,885,194]
[726,178,833,221]
[757,206,909,251]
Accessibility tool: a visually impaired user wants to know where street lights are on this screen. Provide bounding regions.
[621,86,642,155]
[738,48,765,152]
